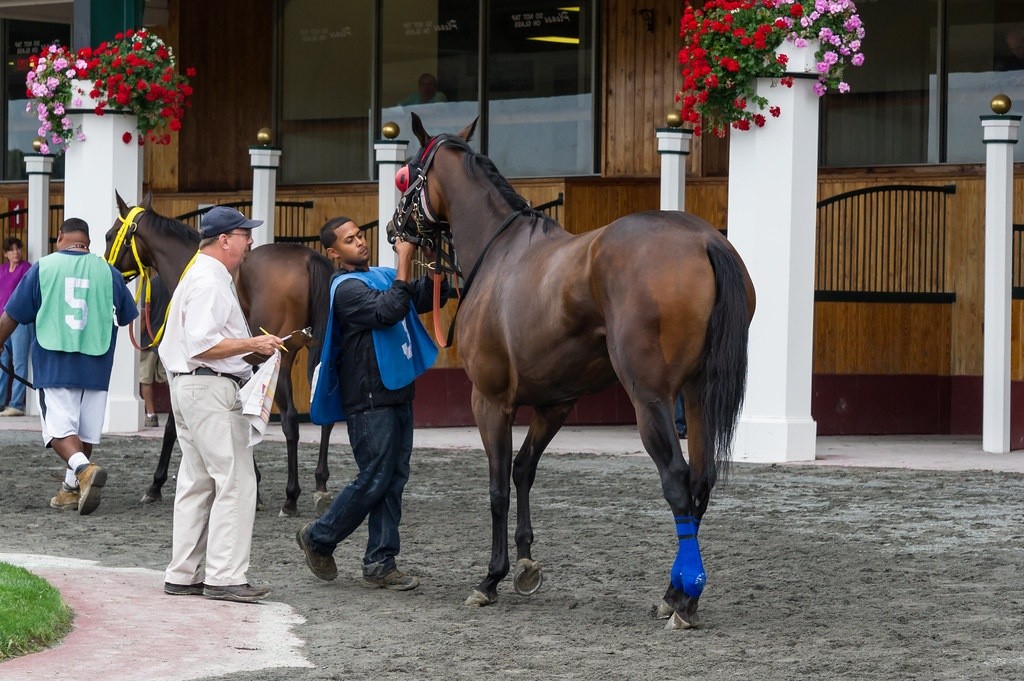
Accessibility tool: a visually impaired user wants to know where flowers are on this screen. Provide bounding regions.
[675,1,865,137]
[26,25,197,155]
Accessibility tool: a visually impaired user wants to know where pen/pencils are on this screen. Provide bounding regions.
[259,326,289,353]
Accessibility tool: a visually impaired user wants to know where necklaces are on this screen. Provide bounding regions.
[66,244,87,250]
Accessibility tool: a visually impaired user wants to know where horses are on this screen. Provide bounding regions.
[386,110,756,628]
[103,189,339,517]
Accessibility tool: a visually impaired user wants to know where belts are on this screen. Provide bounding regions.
[173,367,243,389]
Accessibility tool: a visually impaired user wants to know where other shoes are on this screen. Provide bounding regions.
[0,407,24,416]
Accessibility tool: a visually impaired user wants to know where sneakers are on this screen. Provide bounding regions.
[74,463,107,515]
[144,414,158,427]
[296,523,338,581]
[360,567,420,590]
[164,582,204,594]
[50,481,81,509]
[203,583,270,601]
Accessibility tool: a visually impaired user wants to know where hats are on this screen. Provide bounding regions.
[200,206,264,239]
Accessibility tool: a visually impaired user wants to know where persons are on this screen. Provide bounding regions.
[296,217,450,592]
[157,204,285,601]
[0,237,32,416]
[140,267,168,428]
[0,218,139,514]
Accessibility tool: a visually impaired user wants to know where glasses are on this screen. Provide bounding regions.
[216,230,251,240]
